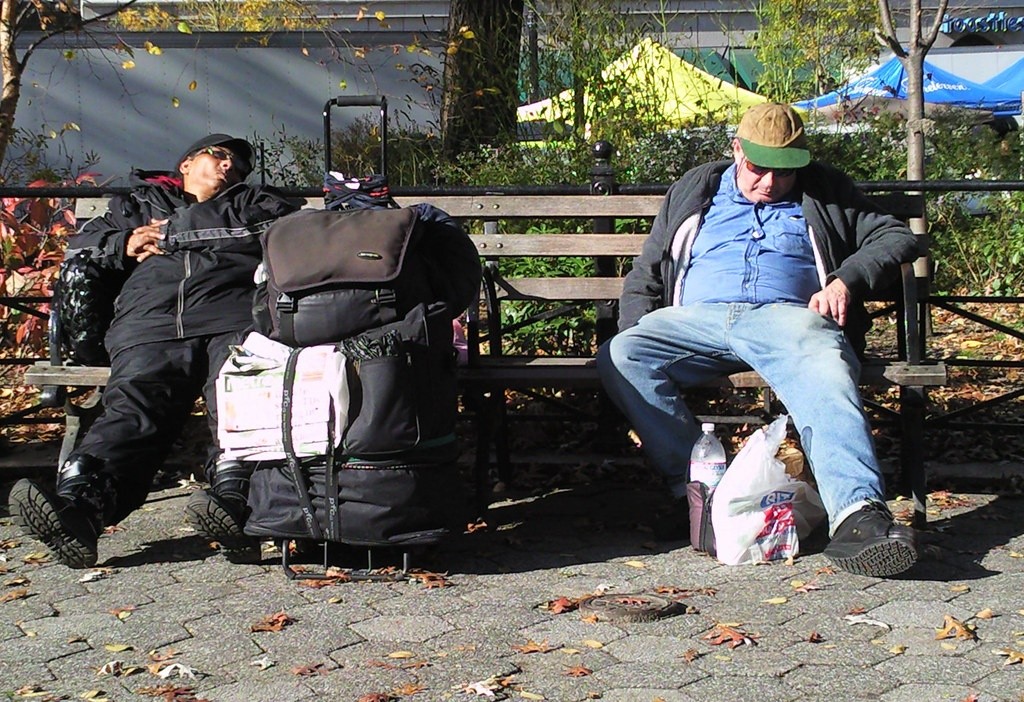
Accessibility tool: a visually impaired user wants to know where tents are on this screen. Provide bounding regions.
[788,50,1024,135]
[516,38,814,149]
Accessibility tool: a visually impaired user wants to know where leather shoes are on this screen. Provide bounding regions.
[825,500,918,577]
[666,496,690,541]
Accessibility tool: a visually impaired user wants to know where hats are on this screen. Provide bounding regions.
[737,102,811,169]
[178,133,256,177]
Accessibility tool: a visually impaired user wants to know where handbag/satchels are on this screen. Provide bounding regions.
[242,205,482,545]
[711,414,826,567]
[53,248,121,366]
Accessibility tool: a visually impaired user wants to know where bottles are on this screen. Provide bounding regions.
[689,423,726,490]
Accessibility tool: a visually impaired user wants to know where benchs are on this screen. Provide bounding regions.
[25,195,947,529]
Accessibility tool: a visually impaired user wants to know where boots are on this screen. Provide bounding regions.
[189,460,261,565]
[8,453,116,570]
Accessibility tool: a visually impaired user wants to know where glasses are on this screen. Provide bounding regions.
[743,158,795,177]
[191,149,244,169]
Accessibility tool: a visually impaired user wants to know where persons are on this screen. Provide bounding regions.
[595,102,922,576]
[10,133,298,569]
